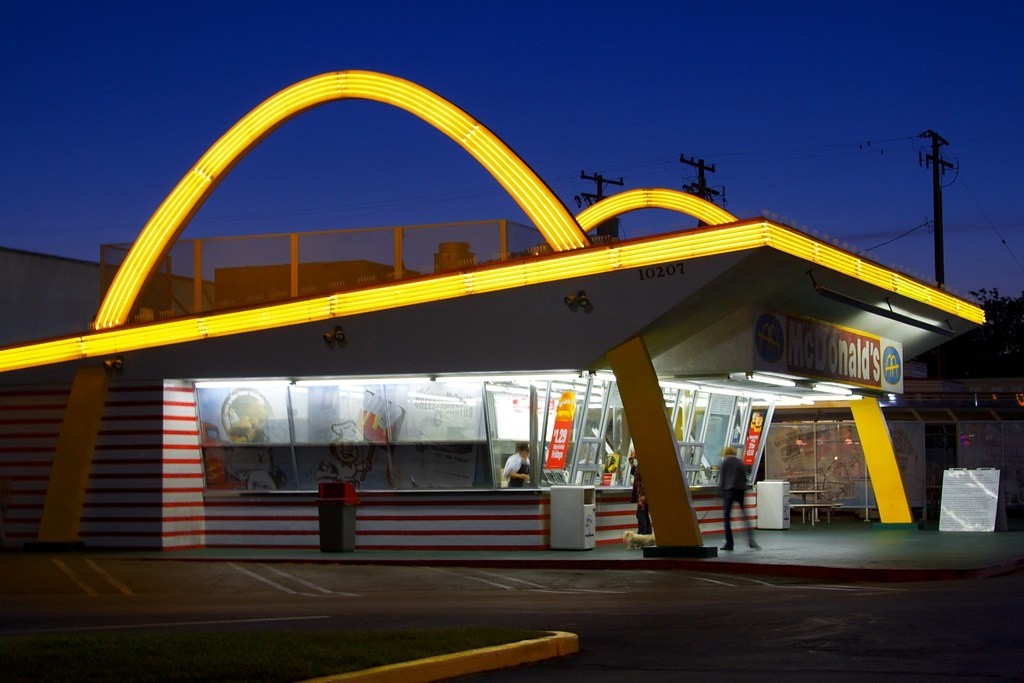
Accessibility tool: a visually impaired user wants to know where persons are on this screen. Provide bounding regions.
[719,447,760,550]
[501,443,530,487]
[605,456,616,473]
[629,451,652,534]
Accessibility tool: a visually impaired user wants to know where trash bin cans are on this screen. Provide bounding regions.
[316,480,361,552]
[550,485,597,550]
[757,481,791,530]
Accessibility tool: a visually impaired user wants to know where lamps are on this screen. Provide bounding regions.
[564,290,589,307]
[322,325,345,342]
[804,268,958,339]
[103,355,125,370]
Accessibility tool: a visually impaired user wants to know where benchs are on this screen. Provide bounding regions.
[789,502,844,524]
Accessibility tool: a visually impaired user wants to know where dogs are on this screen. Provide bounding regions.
[623,531,656,551]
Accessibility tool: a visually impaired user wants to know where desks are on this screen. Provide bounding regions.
[790,490,827,522]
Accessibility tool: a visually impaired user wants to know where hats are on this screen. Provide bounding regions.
[520,442,530,452]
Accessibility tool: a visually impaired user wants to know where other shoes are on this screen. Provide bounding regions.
[720,543,733,551]
[749,542,761,549]
